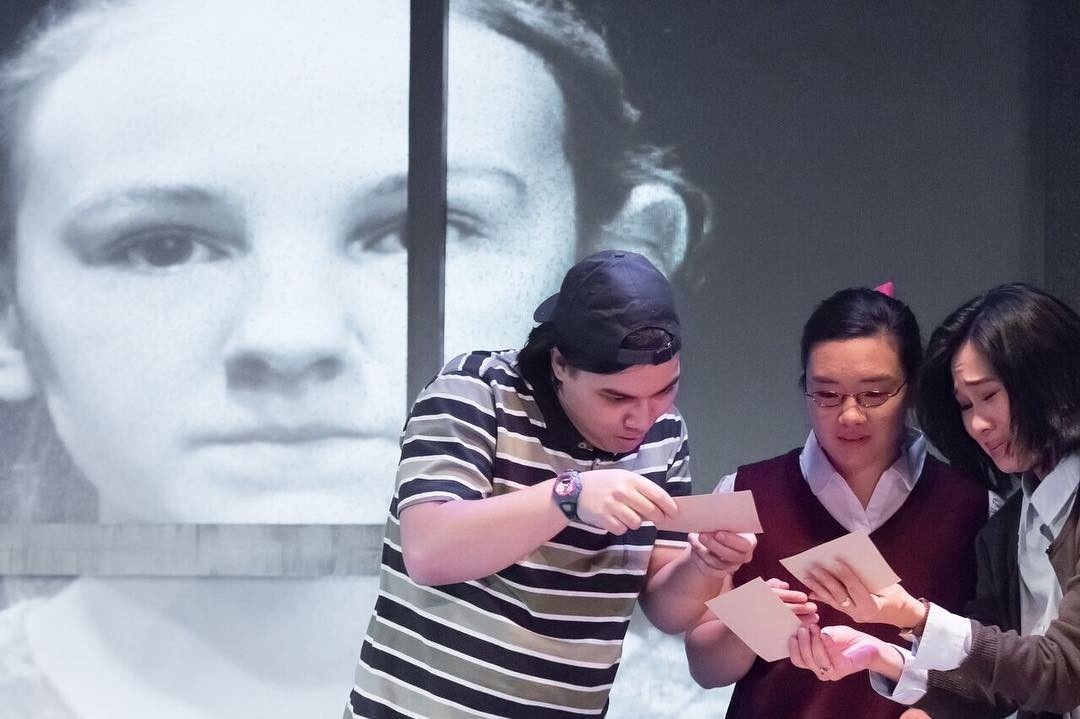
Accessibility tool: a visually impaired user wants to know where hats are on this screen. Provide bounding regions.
[535,250,682,365]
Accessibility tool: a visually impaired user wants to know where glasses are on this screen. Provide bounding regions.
[801,382,906,407]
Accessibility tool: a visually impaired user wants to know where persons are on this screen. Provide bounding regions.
[788,280,1080,719]
[686,287,1005,719]
[0,0,736,719]
[343,250,757,719]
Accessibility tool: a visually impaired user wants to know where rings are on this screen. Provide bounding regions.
[819,664,832,676]
[841,597,851,607]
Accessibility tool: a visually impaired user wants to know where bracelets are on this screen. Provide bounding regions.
[899,598,930,644]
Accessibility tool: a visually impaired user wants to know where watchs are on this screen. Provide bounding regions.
[552,468,582,522]
